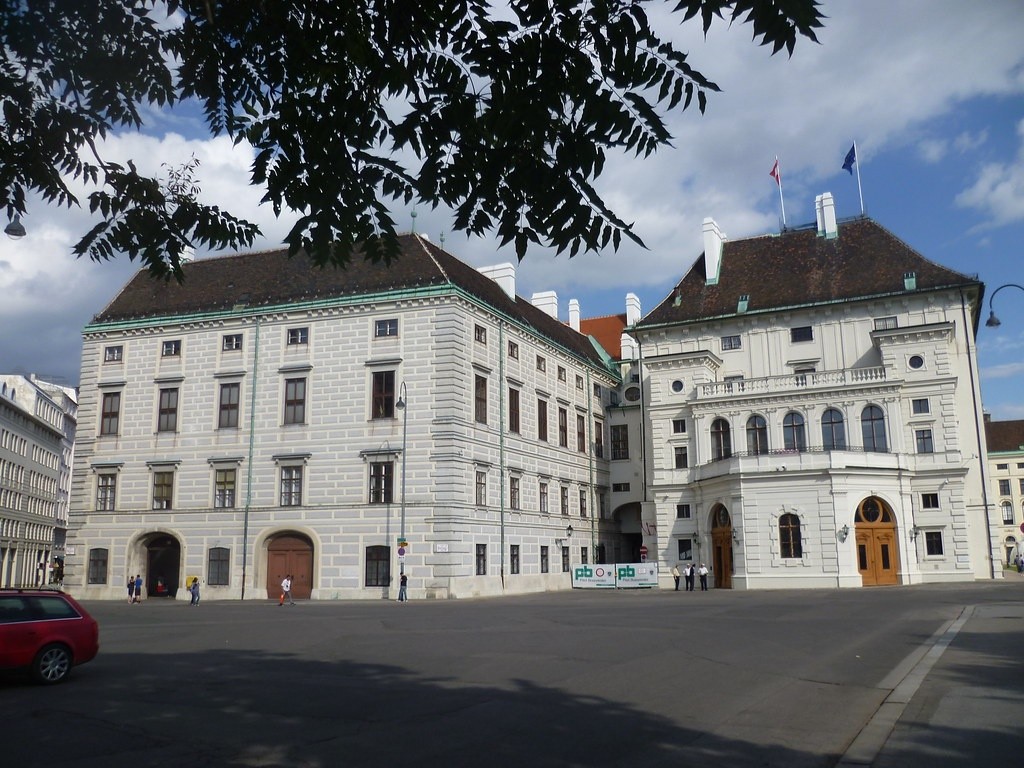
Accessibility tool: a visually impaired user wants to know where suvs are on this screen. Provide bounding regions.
[0,588,100,688]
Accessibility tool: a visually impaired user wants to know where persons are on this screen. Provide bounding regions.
[673,566,681,591]
[698,564,709,591]
[682,564,696,591]
[279,575,296,606]
[1014,554,1024,576]
[189,577,200,607]
[127,575,142,604]
[168,582,174,600]
[395,572,408,602]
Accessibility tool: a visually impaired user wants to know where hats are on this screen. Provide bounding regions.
[701,563,705,566]
[687,563,690,566]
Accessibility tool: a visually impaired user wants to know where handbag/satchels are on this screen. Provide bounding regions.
[280,594,285,604]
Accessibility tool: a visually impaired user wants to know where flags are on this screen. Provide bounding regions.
[769,161,780,185]
[842,145,855,176]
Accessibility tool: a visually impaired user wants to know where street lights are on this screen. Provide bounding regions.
[395,381,408,575]
